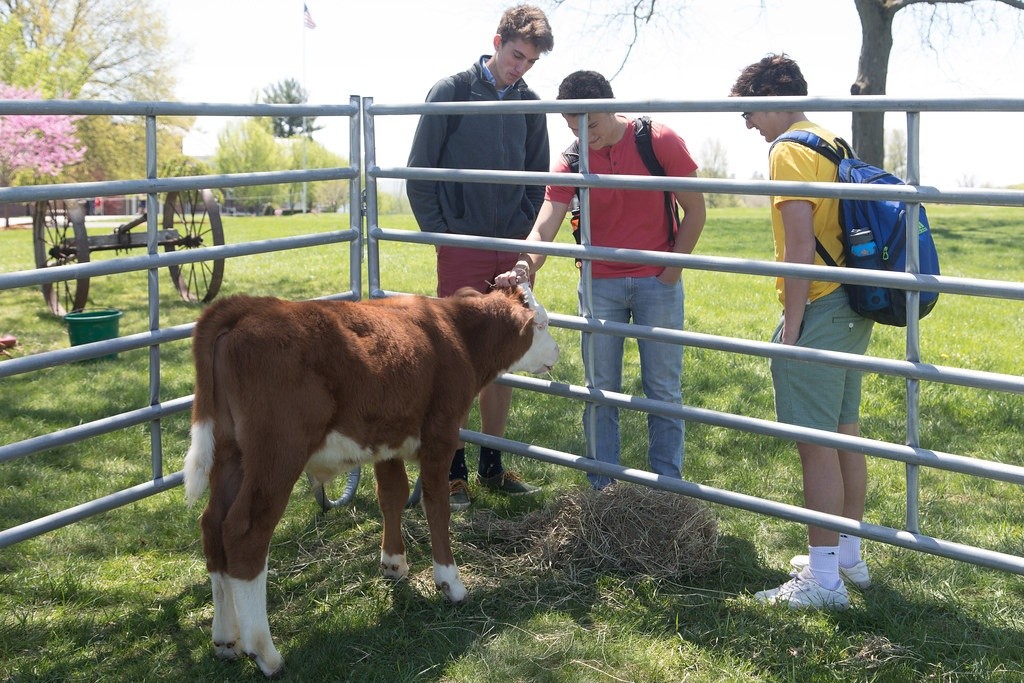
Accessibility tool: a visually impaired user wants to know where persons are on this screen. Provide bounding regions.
[495,70,706,491]
[407,5,553,507]
[730,53,876,611]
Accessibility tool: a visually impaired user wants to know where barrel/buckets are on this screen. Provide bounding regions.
[63,304,124,360]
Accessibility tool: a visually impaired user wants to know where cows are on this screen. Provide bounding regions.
[182,271,559,678]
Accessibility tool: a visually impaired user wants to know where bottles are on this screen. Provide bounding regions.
[849,227,891,315]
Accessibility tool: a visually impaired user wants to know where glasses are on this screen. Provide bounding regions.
[741,111,751,119]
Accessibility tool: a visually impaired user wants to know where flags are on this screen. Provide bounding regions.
[303,3,316,29]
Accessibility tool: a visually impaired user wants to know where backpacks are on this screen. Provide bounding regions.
[768,130,941,326]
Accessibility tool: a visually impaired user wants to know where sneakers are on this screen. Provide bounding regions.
[475,469,542,496]
[753,565,850,610]
[789,554,871,589]
[448,479,472,508]
